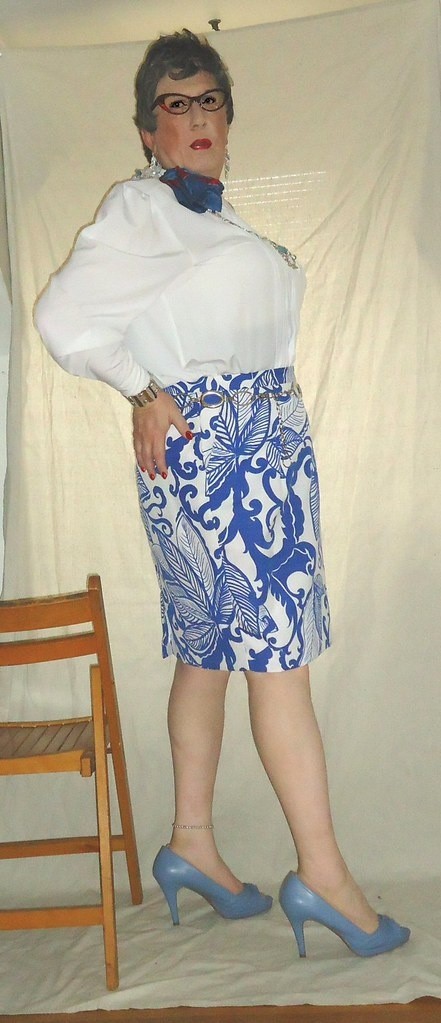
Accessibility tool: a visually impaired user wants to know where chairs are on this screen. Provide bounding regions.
[0,576,143,990]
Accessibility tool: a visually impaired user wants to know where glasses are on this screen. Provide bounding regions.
[150,88,229,115]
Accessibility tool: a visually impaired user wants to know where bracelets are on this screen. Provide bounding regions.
[126,376,160,408]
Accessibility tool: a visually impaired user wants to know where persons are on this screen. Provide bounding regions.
[34,30,410,959]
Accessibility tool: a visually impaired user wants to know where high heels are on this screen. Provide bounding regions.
[280,870,411,958]
[153,845,272,925]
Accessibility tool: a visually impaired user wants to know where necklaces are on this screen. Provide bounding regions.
[206,202,297,270]
